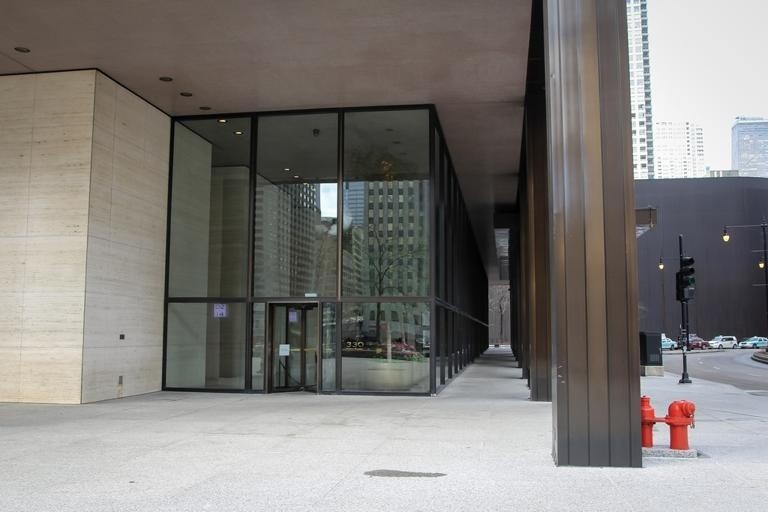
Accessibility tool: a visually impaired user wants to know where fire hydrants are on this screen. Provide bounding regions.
[641,396,695,450]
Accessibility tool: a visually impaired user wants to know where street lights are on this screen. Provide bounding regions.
[658,250,692,351]
[722,216,768,352]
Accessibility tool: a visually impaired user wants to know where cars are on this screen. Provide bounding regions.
[661,333,768,351]
[342,341,430,360]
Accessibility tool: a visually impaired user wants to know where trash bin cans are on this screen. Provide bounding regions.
[639,331,664,376]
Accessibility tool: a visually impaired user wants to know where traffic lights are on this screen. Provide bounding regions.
[676,256,697,302]
